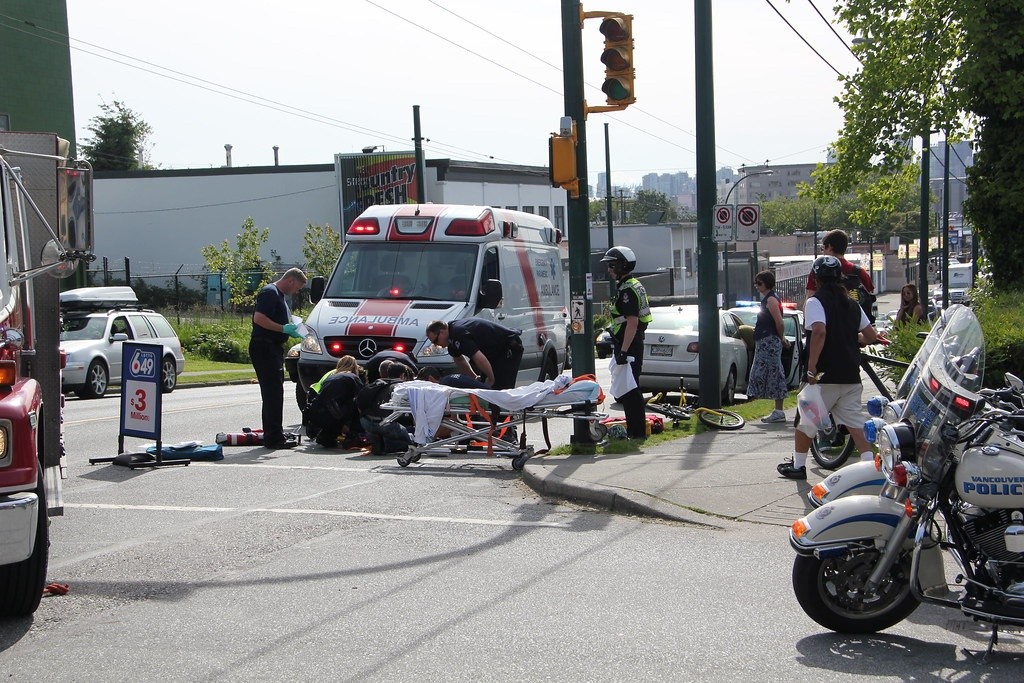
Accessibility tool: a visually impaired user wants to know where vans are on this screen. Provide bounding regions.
[299,202,568,393]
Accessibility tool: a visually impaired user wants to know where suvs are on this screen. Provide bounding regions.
[59,286,185,399]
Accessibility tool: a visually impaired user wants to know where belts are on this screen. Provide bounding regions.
[364,415,381,423]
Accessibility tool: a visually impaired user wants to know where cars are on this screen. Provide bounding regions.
[285,343,301,383]
[641,305,802,406]
[595,321,614,358]
[875,309,898,334]
[928,251,971,267]
[727,301,807,349]
[917,284,942,309]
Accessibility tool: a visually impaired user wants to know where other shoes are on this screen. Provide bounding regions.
[267,441,298,449]
[316,437,338,448]
[831,433,844,448]
[819,440,831,451]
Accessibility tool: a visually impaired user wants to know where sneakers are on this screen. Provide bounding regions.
[761,410,786,423]
[777,456,807,479]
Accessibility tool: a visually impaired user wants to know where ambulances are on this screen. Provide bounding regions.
[0,132,96,615]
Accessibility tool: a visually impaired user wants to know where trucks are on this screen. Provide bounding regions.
[948,262,973,305]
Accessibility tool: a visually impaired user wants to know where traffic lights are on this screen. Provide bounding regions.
[599,14,635,103]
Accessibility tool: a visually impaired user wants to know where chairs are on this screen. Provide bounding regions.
[372,253,412,290]
[447,258,488,299]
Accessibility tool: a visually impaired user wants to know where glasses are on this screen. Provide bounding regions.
[433,332,440,344]
[756,281,764,285]
[608,265,613,269]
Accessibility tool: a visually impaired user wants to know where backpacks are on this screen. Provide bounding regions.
[844,266,876,324]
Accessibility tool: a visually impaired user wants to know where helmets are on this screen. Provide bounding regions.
[812,256,842,278]
[600,246,636,275]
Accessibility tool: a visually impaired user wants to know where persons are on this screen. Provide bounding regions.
[248,268,307,448]
[305,317,524,456]
[894,284,925,331]
[746,270,789,423]
[776,256,876,479]
[802,230,875,452]
[600,247,654,439]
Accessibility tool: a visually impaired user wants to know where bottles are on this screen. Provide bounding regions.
[801,400,827,428]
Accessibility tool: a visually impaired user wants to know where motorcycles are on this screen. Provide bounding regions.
[787,304,1023,665]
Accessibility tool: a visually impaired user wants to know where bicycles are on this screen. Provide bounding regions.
[645,375,745,430]
[810,333,933,468]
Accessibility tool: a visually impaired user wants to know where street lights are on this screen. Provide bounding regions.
[725,170,776,308]
[905,212,920,284]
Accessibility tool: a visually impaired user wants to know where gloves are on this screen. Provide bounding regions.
[283,324,305,339]
[617,351,628,365]
